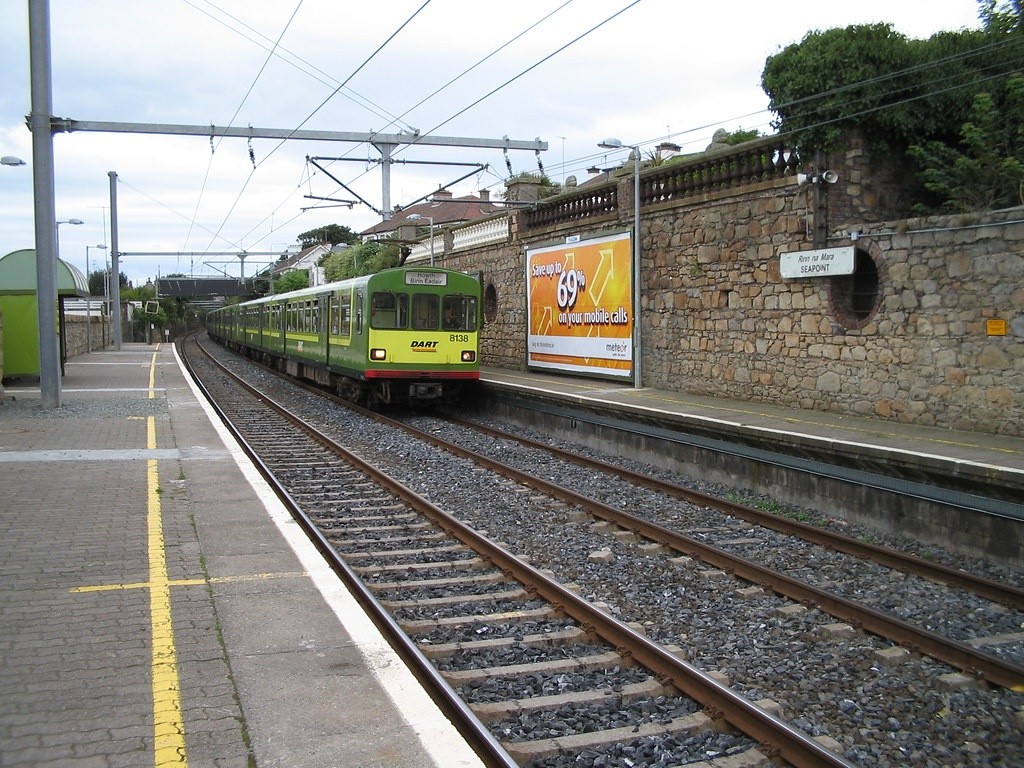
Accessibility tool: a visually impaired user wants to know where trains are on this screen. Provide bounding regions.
[205,267,484,409]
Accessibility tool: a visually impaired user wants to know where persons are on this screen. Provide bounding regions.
[442,309,465,330]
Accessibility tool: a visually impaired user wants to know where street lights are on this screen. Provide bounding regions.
[56,218,85,259]
[406,212,435,267]
[84,243,108,353]
[596,137,643,390]
